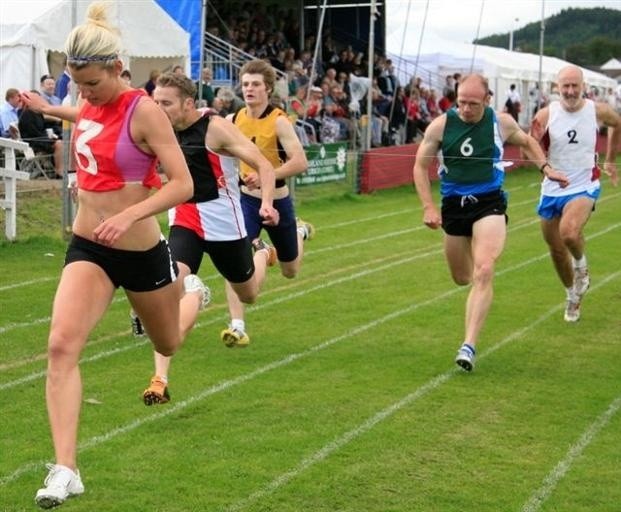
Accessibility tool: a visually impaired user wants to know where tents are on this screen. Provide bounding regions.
[0,0,191,126]
[387,37,617,103]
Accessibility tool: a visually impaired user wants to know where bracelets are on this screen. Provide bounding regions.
[540,163,552,173]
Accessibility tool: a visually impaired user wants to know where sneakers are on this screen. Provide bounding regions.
[143,375,172,407]
[456,347,475,373]
[251,238,277,268]
[184,274,211,309]
[129,310,146,339]
[34,462,86,511]
[565,292,580,324]
[572,256,592,296]
[220,329,250,349]
[295,217,316,242]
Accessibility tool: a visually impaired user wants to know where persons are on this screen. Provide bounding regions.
[122,67,245,119]
[121,59,315,406]
[21,4,210,510]
[413,72,569,373]
[206,0,459,146]
[504,83,560,154]
[0,55,76,179]
[520,67,621,323]
[585,87,621,134]
